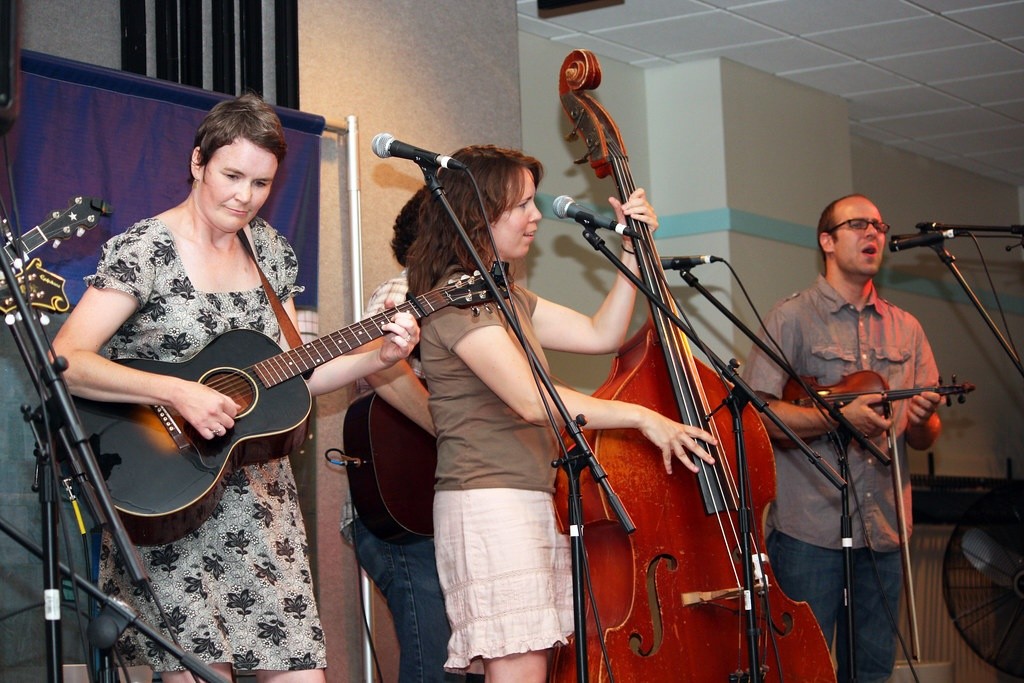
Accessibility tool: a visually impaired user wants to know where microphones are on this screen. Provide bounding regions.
[553,195,642,241]
[658,255,725,269]
[371,133,468,172]
[887,230,953,252]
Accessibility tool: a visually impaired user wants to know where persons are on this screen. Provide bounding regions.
[742,195,945,683]
[46,93,421,683]
[339,145,716,683]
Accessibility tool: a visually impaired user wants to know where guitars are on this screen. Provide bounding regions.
[60,258,516,549]
[341,387,437,544]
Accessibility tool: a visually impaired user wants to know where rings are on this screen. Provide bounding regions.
[213,426,222,435]
[406,335,411,342]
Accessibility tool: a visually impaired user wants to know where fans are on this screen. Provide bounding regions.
[942,480,1024,678]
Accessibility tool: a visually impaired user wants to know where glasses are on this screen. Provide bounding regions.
[827,218,890,234]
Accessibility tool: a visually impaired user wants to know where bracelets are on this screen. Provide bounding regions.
[620,243,635,254]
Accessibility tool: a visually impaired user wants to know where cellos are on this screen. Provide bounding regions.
[554,47,842,683]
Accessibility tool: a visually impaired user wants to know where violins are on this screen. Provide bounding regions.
[770,369,976,453]
